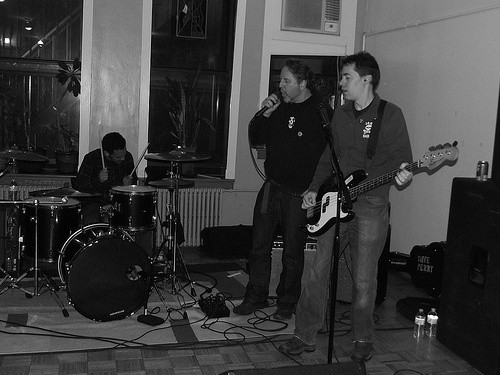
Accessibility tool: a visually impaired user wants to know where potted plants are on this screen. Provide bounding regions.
[164,68,202,178]
[54,125,77,174]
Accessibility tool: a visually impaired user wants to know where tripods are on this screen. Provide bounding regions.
[151,164,198,297]
[0,200,65,296]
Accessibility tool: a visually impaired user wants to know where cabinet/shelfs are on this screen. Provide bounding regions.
[437,178,500,375]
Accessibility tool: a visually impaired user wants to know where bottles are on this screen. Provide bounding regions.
[424,308,438,340]
[413,309,426,339]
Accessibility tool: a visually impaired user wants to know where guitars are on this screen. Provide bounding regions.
[304,140,459,236]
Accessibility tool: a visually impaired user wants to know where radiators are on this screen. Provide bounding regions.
[0,186,224,247]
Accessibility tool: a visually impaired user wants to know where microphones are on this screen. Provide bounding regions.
[316,102,332,131]
[255,89,283,118]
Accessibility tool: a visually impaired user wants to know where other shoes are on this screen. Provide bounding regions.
[274,306,293,319]
[233,298,269,314]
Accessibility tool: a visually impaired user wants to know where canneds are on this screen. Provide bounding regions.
[476,160,489,182]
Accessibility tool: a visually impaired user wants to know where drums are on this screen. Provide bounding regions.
[20,197,82,263]
[57,222,157,322]
[108,185,157,232]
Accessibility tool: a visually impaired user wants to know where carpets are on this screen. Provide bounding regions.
[0,270,297,356]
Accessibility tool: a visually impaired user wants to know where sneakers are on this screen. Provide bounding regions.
[280,336,315,355]
[351,340,373,361]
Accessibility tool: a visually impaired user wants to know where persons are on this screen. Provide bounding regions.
[71,132,138,225]
[278,50,412,360]
[234,58,334,319]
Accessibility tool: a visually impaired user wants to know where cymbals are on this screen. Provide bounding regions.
[148,177,195,189]
[32,187,104,197]
[0,149,49,162]
[146,150,212,161]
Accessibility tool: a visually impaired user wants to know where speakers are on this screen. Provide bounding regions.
[438,176,500,373]
